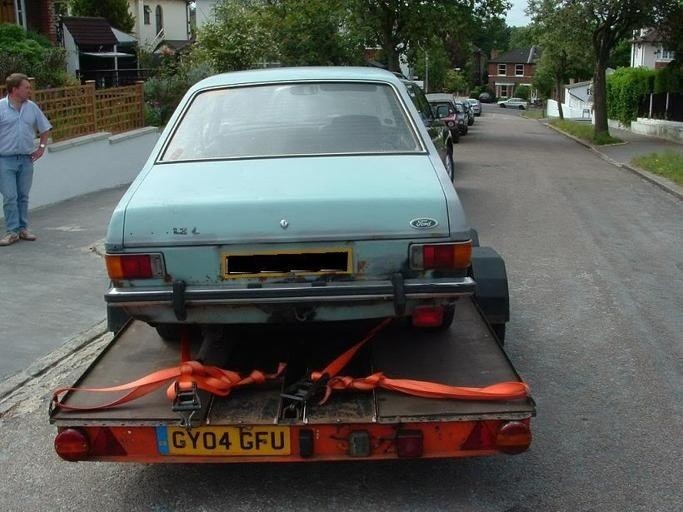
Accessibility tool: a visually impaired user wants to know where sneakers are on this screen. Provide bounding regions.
[0,229,20,246]
[20,229,37,241]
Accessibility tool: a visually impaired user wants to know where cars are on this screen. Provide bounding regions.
[497,98,530,110]
[391,71,454,184]
[479,93,491,103]
[102,65,478,346]
[424,93,481,144]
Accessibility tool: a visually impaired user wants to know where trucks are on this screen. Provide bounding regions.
[47,228,538,465]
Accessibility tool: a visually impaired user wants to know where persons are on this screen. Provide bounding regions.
[0,73,53,247]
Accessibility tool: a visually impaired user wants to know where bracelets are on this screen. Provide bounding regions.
[38,144,44,150]
